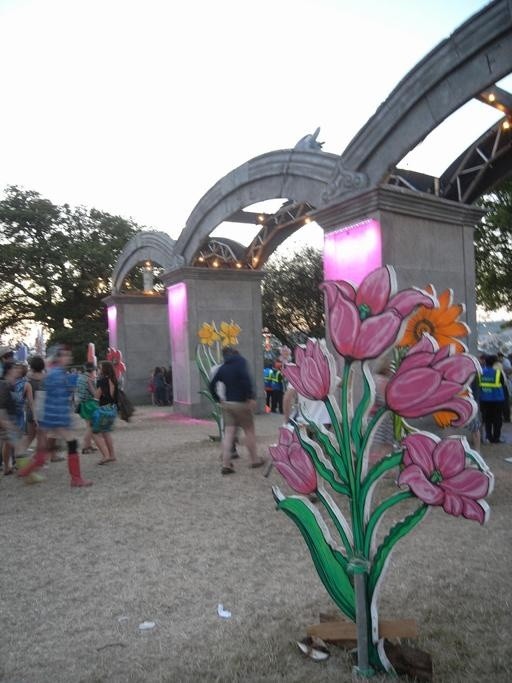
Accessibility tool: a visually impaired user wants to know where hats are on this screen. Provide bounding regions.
[0,345,13,358]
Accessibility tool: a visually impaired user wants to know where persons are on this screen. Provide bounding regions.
[476,350,511,446]
[1,341,123,487]
[264,357,300,425]
[209,347,266,474]
[152,365,174,406]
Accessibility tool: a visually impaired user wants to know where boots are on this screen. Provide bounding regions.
[15,449,51,483]
[67,451,95,488]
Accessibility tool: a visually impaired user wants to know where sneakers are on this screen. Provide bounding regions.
[220,449,268,475]
[13,450,33,459]
[81,444,99,454]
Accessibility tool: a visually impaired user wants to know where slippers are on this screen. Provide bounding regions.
[51,453,67,462]
[97,454,118,466]
[3,466,16,476]
